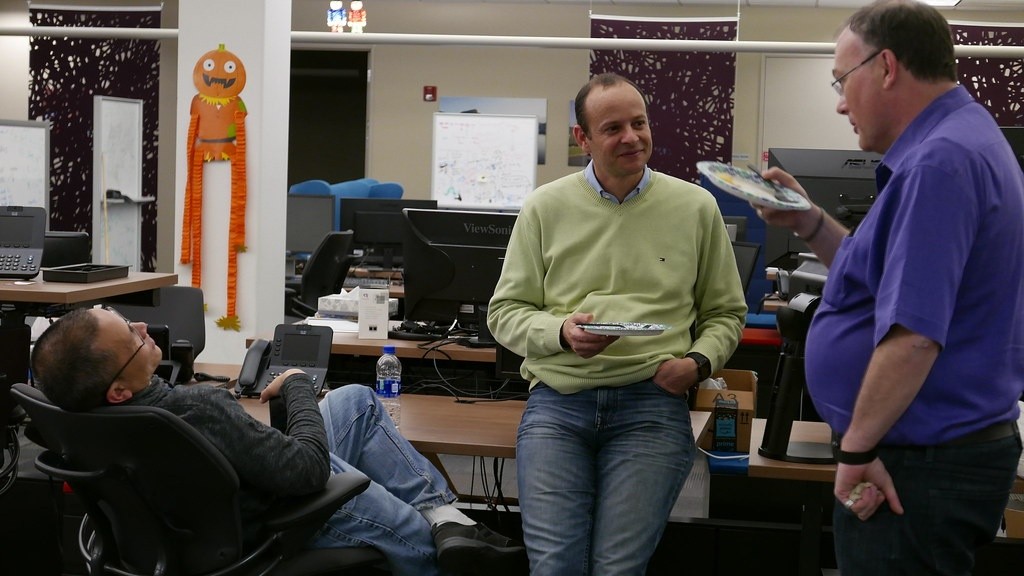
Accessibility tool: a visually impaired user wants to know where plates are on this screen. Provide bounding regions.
[577,322,673,336]
[697,161,812,210]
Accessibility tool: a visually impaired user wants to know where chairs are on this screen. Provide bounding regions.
[8,382,391,576]
[285,230,354,317]
[103,287,205,371]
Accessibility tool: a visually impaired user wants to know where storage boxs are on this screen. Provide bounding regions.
[695,368,757,455]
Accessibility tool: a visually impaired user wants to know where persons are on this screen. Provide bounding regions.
[33,304,526,576]
[486,74,749,576]
[749,0,1024,576]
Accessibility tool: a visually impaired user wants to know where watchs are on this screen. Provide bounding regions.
[686,353,711,381]
[831,435,879,465]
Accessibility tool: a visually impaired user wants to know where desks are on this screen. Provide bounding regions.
[190,315,1024,576]
[1,265,179,371]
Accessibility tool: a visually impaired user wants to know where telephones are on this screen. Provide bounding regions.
[234,323,334,397]
[0,206,47,280]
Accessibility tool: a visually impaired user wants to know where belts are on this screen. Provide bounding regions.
[939,420,1019,447]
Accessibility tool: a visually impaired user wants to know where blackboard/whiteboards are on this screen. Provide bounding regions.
[758,53,863,174]
[0,118,50,232]
[431,112,539,211]
[93,96,142,272]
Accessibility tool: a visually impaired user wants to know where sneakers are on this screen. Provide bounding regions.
[431,521,526,570]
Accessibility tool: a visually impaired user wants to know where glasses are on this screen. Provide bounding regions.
[831,44,903,98]
[103,306,145,406]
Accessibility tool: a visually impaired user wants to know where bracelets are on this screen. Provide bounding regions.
[802,207,825,241]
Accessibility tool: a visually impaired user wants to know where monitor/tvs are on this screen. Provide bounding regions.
[765,148,885,274]
[720,215,761,301]
[285,194,335,253]
[341,197,438,272]
[402,208,523,347]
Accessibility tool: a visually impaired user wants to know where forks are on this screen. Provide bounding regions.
[574,323,652,329]
[746,164,801,202]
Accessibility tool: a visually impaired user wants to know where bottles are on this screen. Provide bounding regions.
[375,346,402,431]
